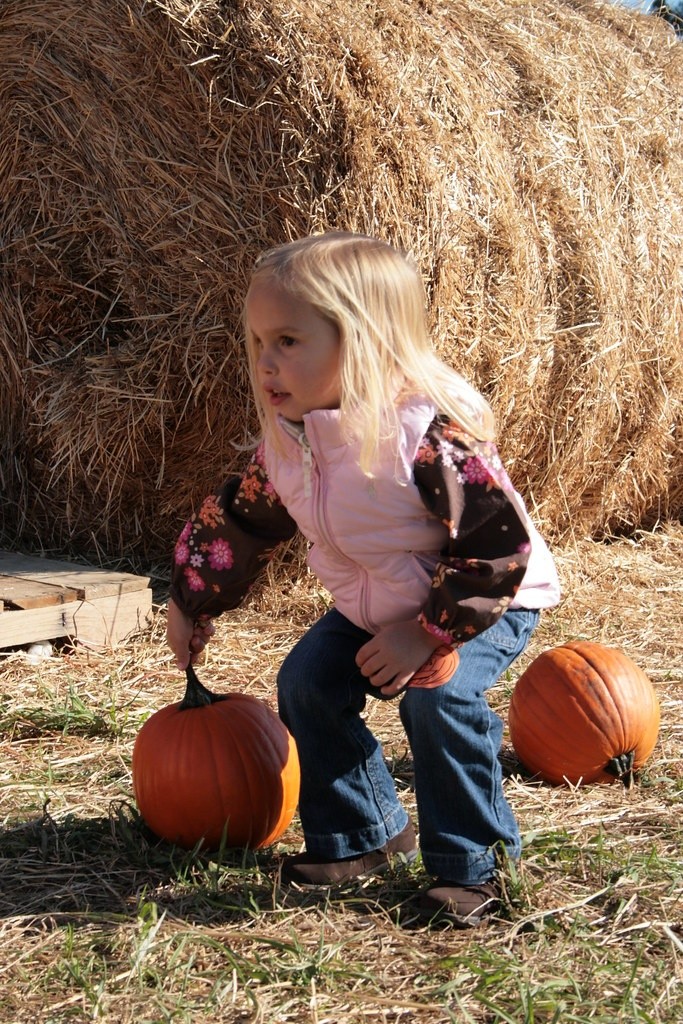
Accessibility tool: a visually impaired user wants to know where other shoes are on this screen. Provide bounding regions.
[414,877,510,927]
[277,814,418,896]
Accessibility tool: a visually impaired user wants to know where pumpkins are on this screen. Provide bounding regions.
[132,660,301,850]
[508,641,660,787]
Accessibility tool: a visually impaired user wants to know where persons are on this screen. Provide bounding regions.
[166,230,561,926]
